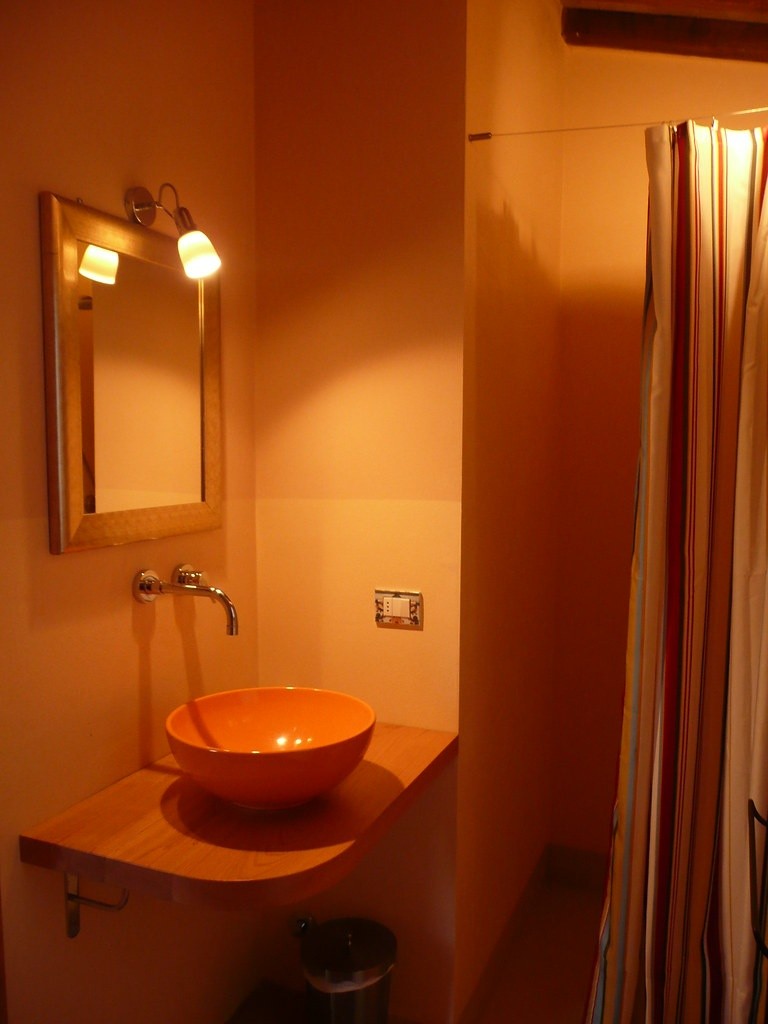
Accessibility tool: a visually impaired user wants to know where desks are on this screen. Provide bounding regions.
[20,725,458,909]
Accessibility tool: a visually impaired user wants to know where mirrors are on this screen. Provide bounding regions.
[37,192,226,554]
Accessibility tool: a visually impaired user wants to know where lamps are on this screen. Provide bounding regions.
[124,181,221,280]
[79,243,118,285]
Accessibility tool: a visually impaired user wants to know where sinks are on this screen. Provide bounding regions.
[164,685,376,811]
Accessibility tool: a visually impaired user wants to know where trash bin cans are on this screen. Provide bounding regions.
[304,917,402,1024]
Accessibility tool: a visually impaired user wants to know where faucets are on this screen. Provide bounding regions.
[132,563,239,637]
